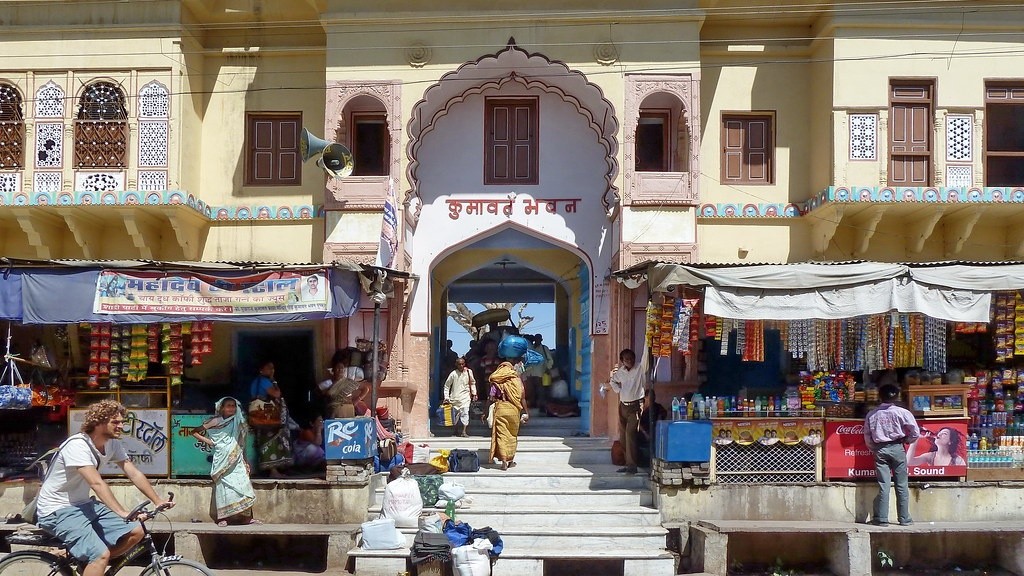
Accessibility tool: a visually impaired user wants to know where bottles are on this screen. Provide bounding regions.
[672,393,787,421]
[784,385,801,417]
[905,370,941,386]
[446,499,455,521]
[966,433,1024,467]
[920,429,937,439]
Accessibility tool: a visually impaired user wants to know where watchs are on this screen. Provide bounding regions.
[273,381,277,383]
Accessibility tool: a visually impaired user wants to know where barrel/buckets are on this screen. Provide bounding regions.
[542,373,552,386]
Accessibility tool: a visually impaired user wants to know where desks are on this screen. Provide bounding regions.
[709,416,967,482]
[171,414,256,481]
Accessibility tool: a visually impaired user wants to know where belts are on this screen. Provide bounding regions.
[622,400,642,406]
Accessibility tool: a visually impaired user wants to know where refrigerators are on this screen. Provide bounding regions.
[171,412,256,478]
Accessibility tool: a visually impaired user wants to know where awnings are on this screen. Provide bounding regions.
[1,255,418,478]
[611,259,1024,462]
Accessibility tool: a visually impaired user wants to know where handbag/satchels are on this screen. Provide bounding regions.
[249,397,287,428]
[435,400,455,426]
[470,401,483,415]
[0,360,77,409]
[449,448,479,472]
[22,490,38,525]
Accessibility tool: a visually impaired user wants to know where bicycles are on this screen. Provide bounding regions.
[0,491,213,576]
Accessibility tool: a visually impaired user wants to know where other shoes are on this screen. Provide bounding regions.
[616,467,638,477]
[867,519,889,527]
[900,519,914,526]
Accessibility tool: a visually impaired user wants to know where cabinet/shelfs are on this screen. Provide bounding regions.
[67,376,181,479]
[904,385,969,418]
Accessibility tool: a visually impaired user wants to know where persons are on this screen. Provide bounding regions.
[250,361,280,401]
[487,357,529,470]
[713,429,733,445]
[317,363,369,418]
[906,427,965,466]
[610,336,649,473]
[189,396,255,527]
[304,275,322,300]
[759,429,779,445]
[864,385,920,525]
[446,333,554,412]
[803,429,822,445]
[636,389,667,465]
[440,357,478,438]
[36,398,176,576]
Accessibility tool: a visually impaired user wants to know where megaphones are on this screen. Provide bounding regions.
[318,142,353,179]
[300,127,333,163]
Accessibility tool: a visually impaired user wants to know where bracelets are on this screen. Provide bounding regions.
[352,396,362,406]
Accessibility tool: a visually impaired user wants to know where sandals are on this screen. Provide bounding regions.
[218,520,227,526]
[242,518,255,524]
